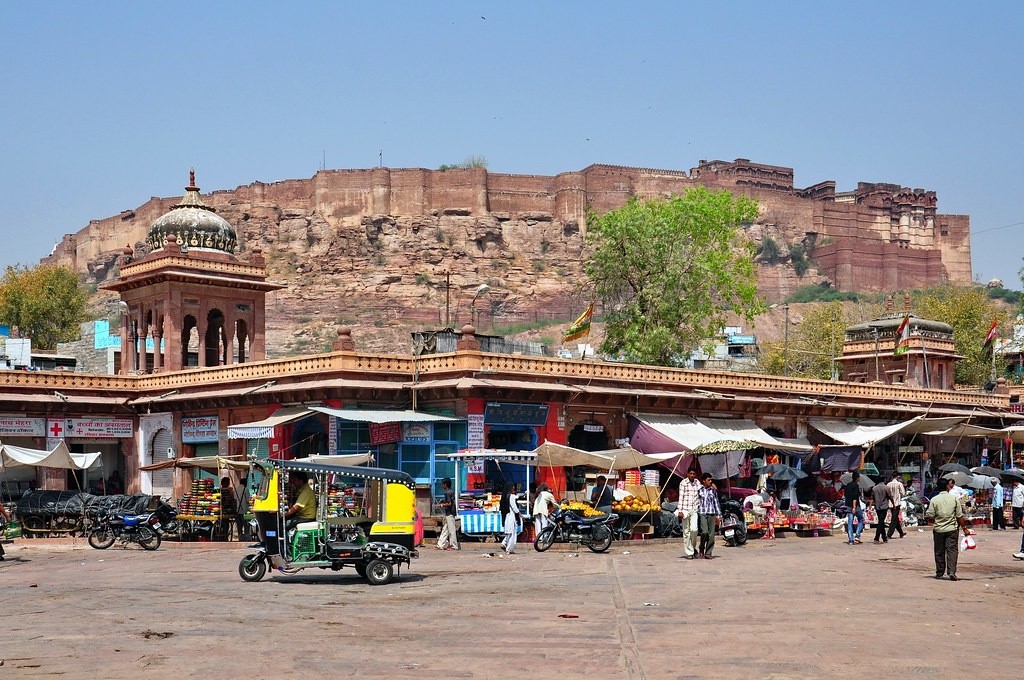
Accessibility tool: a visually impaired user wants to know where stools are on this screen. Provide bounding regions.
[297,521,324,531]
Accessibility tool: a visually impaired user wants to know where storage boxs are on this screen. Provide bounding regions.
[633,523,655,539]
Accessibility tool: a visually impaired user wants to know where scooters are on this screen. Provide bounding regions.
[718,495,747,547]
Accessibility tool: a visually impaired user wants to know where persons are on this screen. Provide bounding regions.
[96,478,107,495]
[220,477,230,541]
[588,475,615,547]
[533,483,560,544]
[414,507,423,547]
[759,488,775,540]
[844,470,907,544]
[22,480,42,498]
[925,478,1024,581]
[677,465,723,559]
[106,469,120,494]
[279,470,317,562]
[234,477,250,541]
[433,477,458,551]
[499,480,525,555]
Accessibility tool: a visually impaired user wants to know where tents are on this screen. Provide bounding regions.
[0,438,106,507]
[500,438,686,519]
[137,454,251,541]
[287,450,375,518]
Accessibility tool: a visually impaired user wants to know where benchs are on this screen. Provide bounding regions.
[370,520,417,536]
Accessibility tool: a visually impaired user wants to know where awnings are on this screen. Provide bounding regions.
[306,407,468,424]
[806,415,1024,466]
[227,406,321,439]
[627,413,761,500]
[773,436,815,455]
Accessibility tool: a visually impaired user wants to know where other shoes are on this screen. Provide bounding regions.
[885,537,890,543]
[1013,551,1024,560]
[687,555,692,559]
[887,534,892,539]
[854,538,863,544]
[447,547,458,551]
[874,541,884,544]
[699,548,704,557]
[433,547,442,550]
[705,554,713,559]
[900,533,906,538]
[501,544,506,552]
[849,540,854,545]
[935,573,943,579]
[267,548,282,557]
[693,549,698,559]
[506,551,516,554]
[949,571,957,581]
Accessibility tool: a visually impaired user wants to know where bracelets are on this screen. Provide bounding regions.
[440,503,444,508]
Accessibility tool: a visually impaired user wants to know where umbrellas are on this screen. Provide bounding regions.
[755,462,809,480]
[937,463,1024,489]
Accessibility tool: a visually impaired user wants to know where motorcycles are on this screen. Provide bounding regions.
[534,508,619,553]
[88,504,165,550]
[239,469,416,585]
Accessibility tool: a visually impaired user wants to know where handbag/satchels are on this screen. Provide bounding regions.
[961,534,976,551]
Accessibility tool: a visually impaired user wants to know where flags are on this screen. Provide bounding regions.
[564,304,591,344]
[978,320,997,363]
[894,313,909,356]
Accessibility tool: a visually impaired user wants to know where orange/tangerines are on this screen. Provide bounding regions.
[562,502,603,516]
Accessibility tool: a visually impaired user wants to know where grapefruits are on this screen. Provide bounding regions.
[612,495,662,512]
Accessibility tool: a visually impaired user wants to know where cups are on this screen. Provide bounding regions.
[488,493,491,501]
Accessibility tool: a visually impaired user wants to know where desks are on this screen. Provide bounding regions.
[460,513,503,540]
[178,513,235,542]
